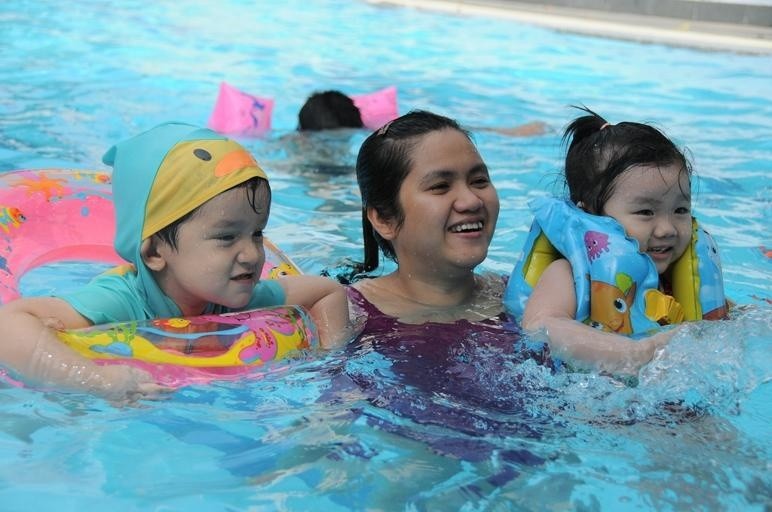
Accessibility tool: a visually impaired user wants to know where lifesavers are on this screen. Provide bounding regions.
[0,168,320,395]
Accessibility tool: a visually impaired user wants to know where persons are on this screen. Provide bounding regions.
[256,84,551,233]
[0,119,351,445]
[521,93,772,511]
[238,104,597,512]
